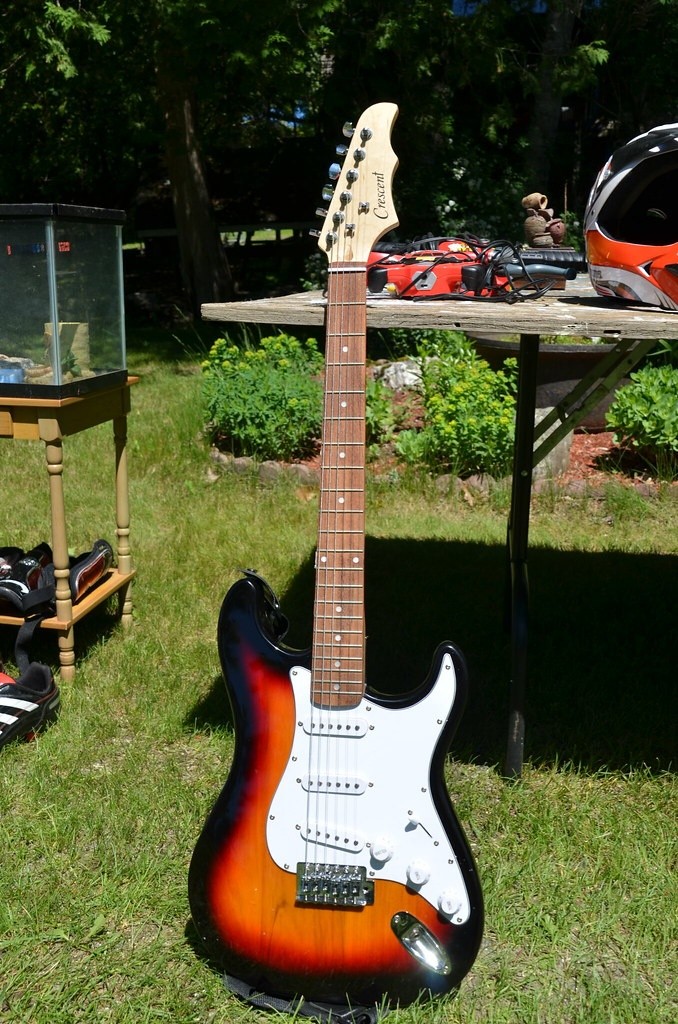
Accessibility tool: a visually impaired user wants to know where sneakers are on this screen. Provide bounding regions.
[0,661,59,747]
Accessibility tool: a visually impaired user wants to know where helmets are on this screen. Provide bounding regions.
[583,123,677,311]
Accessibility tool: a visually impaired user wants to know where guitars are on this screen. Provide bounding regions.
[185,99,487,1008]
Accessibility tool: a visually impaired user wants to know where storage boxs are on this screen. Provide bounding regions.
[0,202,127,398]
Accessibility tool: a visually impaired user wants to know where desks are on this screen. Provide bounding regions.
[0,376,140,685]
[199,287,678,783]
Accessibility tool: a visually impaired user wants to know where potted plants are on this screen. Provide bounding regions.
[467,331,646,432]
